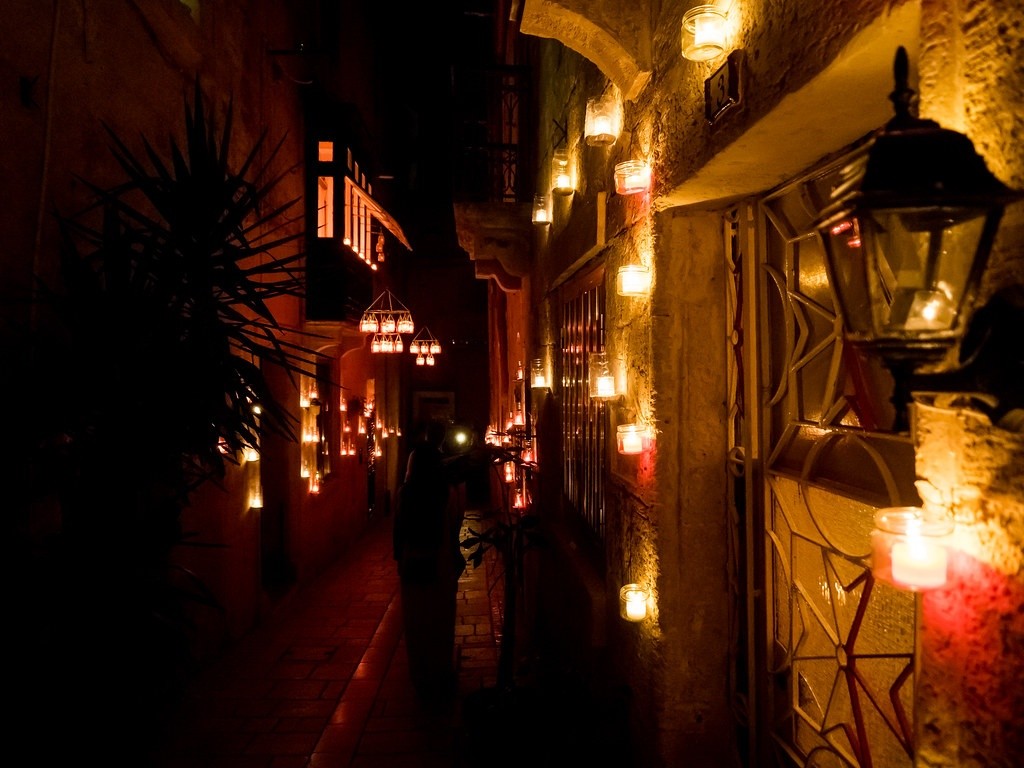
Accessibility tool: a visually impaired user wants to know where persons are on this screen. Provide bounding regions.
[391,422,471,732]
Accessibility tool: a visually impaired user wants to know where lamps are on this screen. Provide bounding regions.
[808,44,1024,437]
[530,357,551,390]
[551,148,577,196]
[588,352,624,407]
[615,424,653,456]
[583,95,621,147]
[356,286,414,353]
[243,395,414,516]
[867,503,960,590]
[620,584,651,620]
[617,265,653,296]
[409,325,442,366]
[614,160,649,194]
[532,193,552,226]
[681,4,728,60]
[484,402,535,509]
